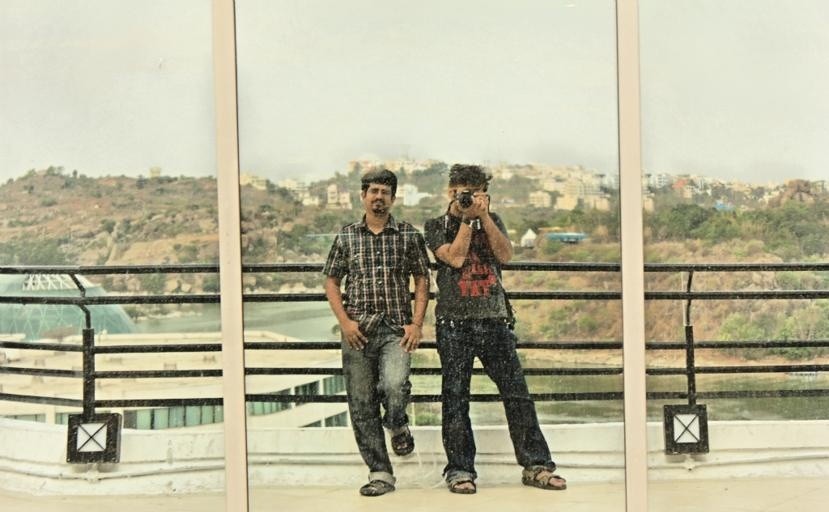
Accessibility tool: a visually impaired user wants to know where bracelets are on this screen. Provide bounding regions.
[461,217,475,228]
[411,319,423,327]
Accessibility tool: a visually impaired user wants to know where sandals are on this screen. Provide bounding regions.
[391,424,415,456]
[360,480,395,496]
[446,470,476,493]
[521,466,567,490]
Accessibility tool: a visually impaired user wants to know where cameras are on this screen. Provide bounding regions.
[453,189,474,209]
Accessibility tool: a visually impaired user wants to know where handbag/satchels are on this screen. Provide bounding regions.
[503,287,516,330]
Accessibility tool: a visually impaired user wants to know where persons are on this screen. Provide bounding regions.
[423,164,569,493]
[322,168,433,497]
